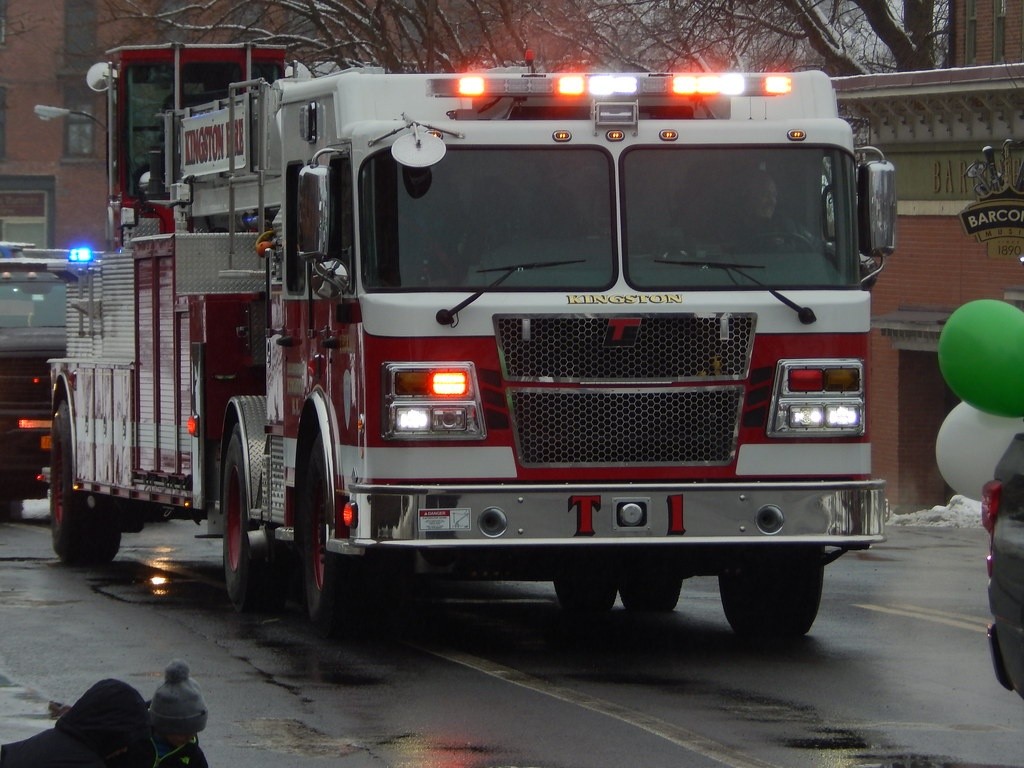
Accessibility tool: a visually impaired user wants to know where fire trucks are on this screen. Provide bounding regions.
[0,242,105,517]
[45,44,899,643]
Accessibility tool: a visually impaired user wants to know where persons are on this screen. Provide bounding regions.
[0,658,209,768]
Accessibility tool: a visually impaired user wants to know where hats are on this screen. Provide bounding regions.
[146,657,209,732]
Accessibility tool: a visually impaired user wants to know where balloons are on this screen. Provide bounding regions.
[936,300,1024,501]
